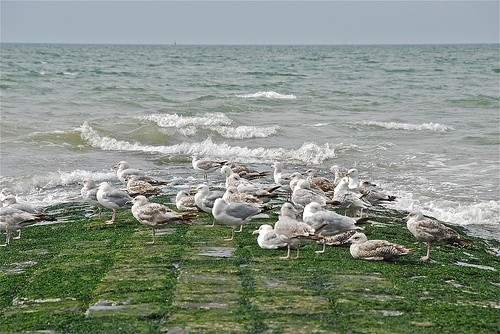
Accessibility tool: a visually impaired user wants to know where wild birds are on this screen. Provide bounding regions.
[0,159,399,251]
[400,205,481,260]
[274,202,316,262]
[341,232,407,261]
[306,200,374,257]
